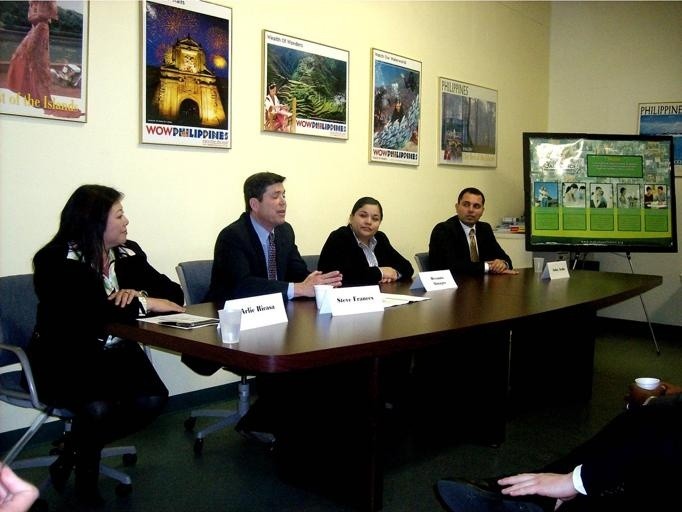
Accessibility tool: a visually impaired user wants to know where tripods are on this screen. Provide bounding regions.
[572,252,660,354]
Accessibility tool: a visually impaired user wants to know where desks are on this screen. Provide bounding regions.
[112,267,662,512]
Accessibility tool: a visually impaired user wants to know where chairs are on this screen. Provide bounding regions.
[302,255,321,272]
[414,252,429,271]
[175,261,276,454]
[0,273,136,499]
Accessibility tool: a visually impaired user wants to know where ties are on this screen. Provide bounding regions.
[268,233,279,281]
[469,230,480,262]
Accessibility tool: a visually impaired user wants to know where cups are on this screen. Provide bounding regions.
[533,257,544,273]
[635,377,660,391]
[218,308,241,344]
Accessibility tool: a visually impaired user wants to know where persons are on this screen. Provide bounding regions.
[0,463,49,512]
[390,100,405,124]
[20,185,187,508]
[591,187,607,208]
[429,188,519,275]
[201,173,344,444]
[624,381,682,405]
[654,186,666,205]
[7,1,85,118]
[644,187,653,208]
[564,184,578,204]
[619,187,626,207]
[438,394,682,512]
[265,82,288,130]
[538,185,550,207]
[318,197,413,285]
[578,186,586,204]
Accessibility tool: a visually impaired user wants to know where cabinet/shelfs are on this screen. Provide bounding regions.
[494,229,570,269]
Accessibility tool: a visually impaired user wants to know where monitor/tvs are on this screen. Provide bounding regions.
[522,130,678,254]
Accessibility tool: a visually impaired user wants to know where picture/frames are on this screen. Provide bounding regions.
[138,0,233,149]
[0,0,90,123]
[368,48,423,166]
[261,29,349,140]
[437,76,498,168]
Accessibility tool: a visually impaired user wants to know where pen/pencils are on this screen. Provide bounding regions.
[158,322,194,327]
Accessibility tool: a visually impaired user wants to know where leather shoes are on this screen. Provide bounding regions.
[234,427,279,445]
[435,476,551,512]
[47,460,66,496]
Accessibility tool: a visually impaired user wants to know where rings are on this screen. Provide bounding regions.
[124,291,129,294]
